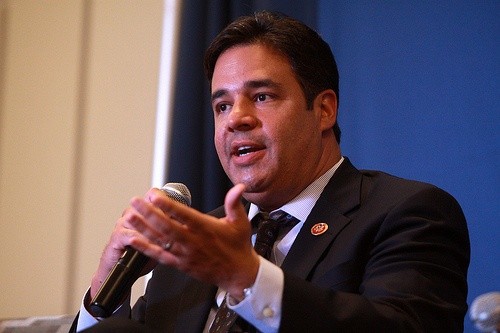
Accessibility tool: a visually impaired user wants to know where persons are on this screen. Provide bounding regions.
[66,8,471,333]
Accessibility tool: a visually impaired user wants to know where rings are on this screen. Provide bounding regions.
[163,238,174,251]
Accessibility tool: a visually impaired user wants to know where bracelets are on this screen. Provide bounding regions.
[242,284,254,300]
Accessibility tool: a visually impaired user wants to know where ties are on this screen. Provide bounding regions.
[209,210,301,332]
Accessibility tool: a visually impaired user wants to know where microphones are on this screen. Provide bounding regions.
[89,181,193,320]
[468,292,500,333]
